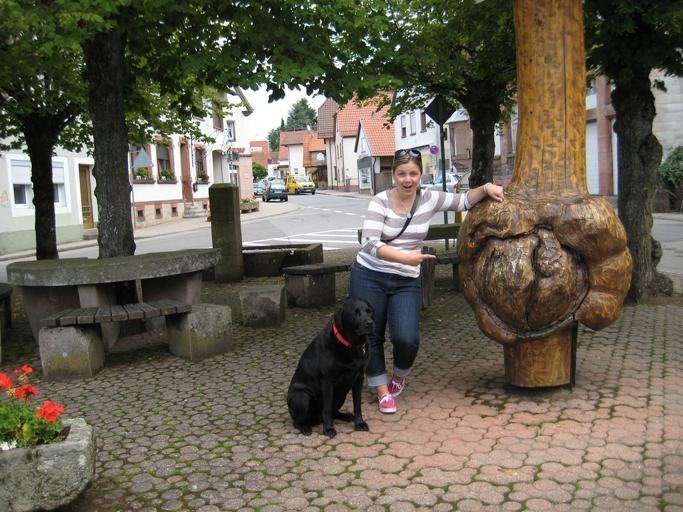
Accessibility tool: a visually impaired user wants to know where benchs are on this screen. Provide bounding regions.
[282,250,460,310]
[38,298,233,379]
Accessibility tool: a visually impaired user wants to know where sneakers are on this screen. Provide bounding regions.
[377,374,406,414]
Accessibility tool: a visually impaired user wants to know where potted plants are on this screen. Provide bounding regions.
[137,167,150,180]
[198,170,209,183]
[161,169,174,180]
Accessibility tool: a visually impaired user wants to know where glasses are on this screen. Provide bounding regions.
[395,149,421,159]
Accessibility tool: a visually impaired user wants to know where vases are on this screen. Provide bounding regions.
[0,418,98,512]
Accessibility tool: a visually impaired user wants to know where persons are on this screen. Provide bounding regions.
[346,149,505,414]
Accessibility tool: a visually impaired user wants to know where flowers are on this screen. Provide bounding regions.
[0,364,64,446]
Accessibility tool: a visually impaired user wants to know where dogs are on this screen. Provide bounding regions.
[285,296,376,439]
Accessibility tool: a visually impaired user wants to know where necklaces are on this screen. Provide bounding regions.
[395,191,415,219]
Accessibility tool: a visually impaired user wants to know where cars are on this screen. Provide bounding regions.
[424,172,471,193]
[252,174,316,202]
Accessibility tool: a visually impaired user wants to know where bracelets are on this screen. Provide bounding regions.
[483,183,491,194]
